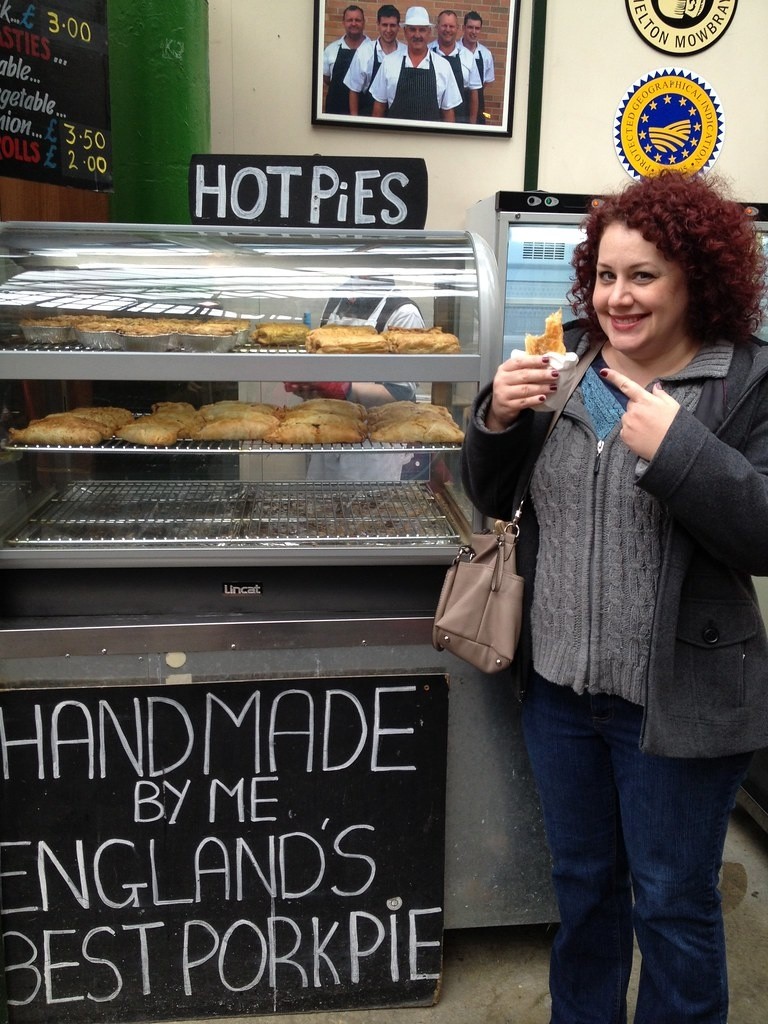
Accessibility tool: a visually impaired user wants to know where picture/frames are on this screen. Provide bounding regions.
[311,0,521,139]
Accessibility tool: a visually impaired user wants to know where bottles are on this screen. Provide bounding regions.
[303,312,311,330]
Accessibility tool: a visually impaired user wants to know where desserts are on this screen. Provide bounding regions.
[19,313,253,354]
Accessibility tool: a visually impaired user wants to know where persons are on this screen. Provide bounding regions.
[462,169,768,1024]
[284,295,425,482]
[322,4,495,124]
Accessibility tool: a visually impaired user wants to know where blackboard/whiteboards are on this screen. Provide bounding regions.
[0,0,115,192]
[188,154,429,230]
[0,671,450,1023]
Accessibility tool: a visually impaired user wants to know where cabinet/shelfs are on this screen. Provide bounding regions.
[0,340,478,568]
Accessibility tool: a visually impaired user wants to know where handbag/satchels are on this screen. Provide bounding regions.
[434,341,605,674]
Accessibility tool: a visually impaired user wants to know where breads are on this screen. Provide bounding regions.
[8,398,467,451]
[252,321,462,355]
[522,307,568,357]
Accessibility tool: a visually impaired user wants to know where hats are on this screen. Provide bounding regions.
[398,6,433,27]
